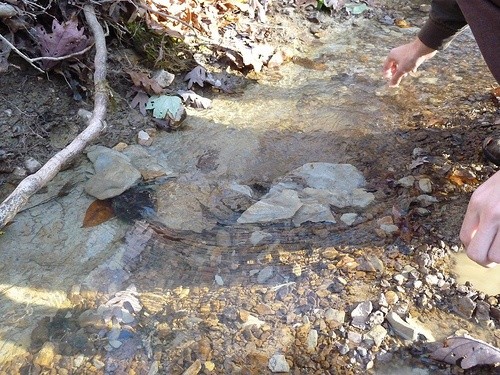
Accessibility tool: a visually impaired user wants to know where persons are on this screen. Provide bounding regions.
[378,1,500,268]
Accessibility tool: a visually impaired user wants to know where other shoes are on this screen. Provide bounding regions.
[483,132,500,167]
